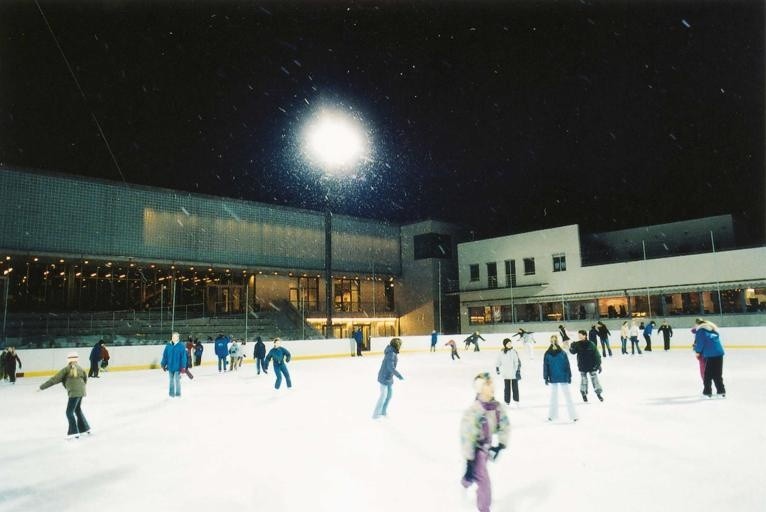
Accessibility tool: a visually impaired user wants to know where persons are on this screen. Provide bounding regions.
[494,304,673,422]
[354,328,363,356]
[88,340,110,378]
[1,346,22,382]
[692,318,726,399]
[206,332,247,371]
[429,329,487,360]
[162,331,204,397]
[35,351,93,439]
[373,338,403,419]
[457,371,511,510]
[254,337,291,390]
